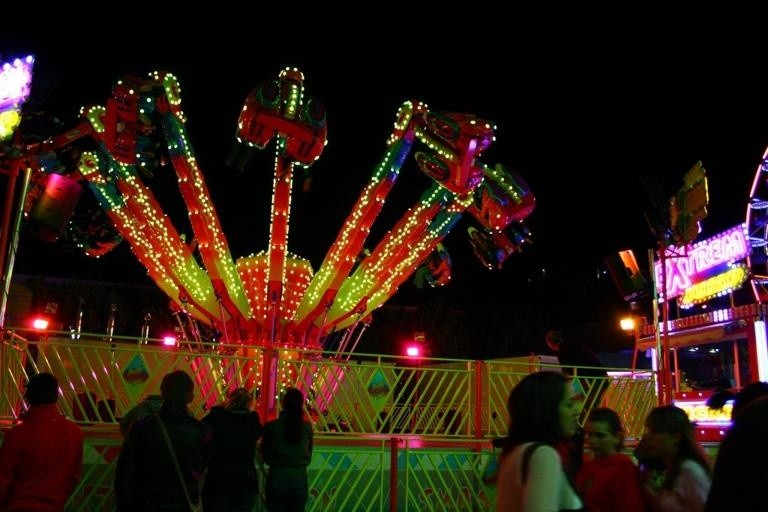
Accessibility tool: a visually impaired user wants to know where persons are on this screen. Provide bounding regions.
[701,378,767,512]
[119,372,172,439]
[480,369,715,512]
[199,385,264,510]
[110,368,212,510]
[260,387,314,512]
[0,370,84,509]
